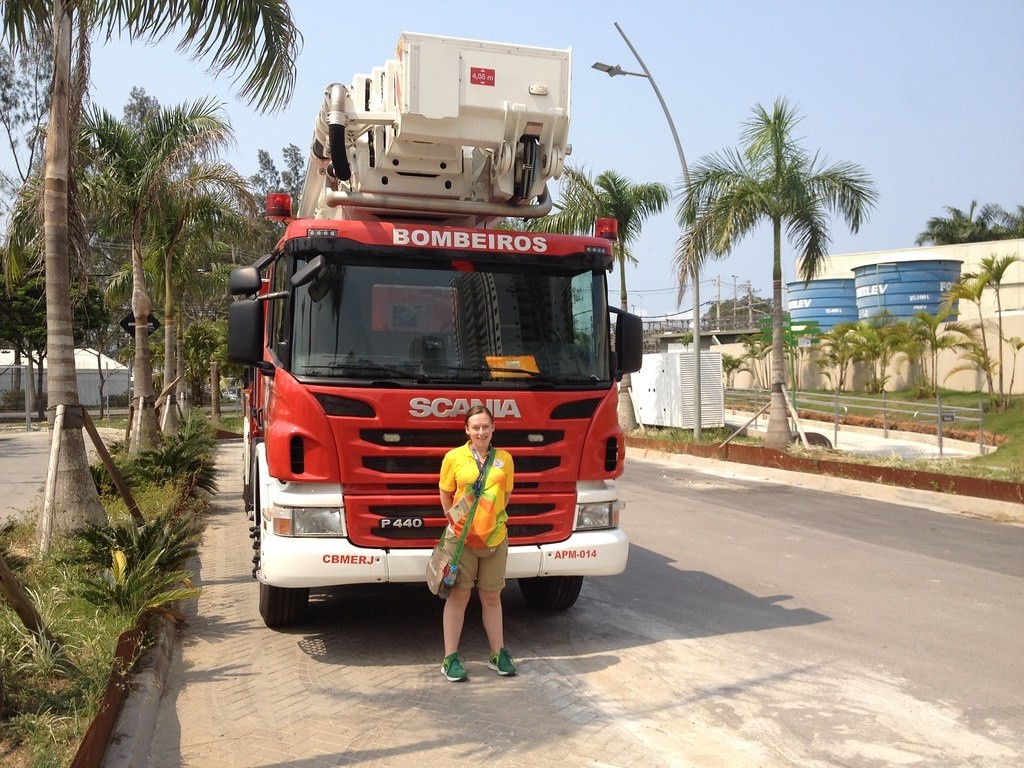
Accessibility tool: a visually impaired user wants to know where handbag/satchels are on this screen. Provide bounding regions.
[425,544,459,599]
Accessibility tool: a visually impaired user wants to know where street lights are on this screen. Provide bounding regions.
[590,22,701,445]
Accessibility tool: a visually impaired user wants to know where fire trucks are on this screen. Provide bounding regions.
[225,30,644,631]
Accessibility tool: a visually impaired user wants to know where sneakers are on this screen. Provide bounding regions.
[441,657,468,681]
[488,648,517,676]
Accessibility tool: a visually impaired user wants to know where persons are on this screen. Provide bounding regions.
[440,404,516,683]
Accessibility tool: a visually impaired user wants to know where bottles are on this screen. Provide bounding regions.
[438,565,457,599]
